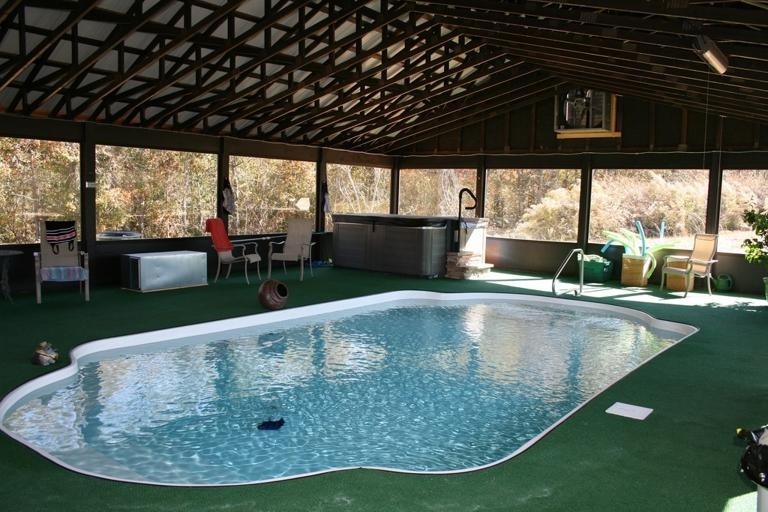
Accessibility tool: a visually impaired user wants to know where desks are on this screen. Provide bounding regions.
[121,251,209,294]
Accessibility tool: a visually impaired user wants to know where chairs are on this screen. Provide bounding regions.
[267,218,317,283]
[660,233,719,298]
[206,219,262,285]
[33,220,90,304]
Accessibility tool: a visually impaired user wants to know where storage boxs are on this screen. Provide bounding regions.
[578,253,614,282]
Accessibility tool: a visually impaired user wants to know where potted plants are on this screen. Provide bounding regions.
[741,205,768,299]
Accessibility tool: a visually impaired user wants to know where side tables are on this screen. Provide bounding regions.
[0,250,24,304]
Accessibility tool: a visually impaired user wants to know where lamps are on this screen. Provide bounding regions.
[692,34,729,75]
